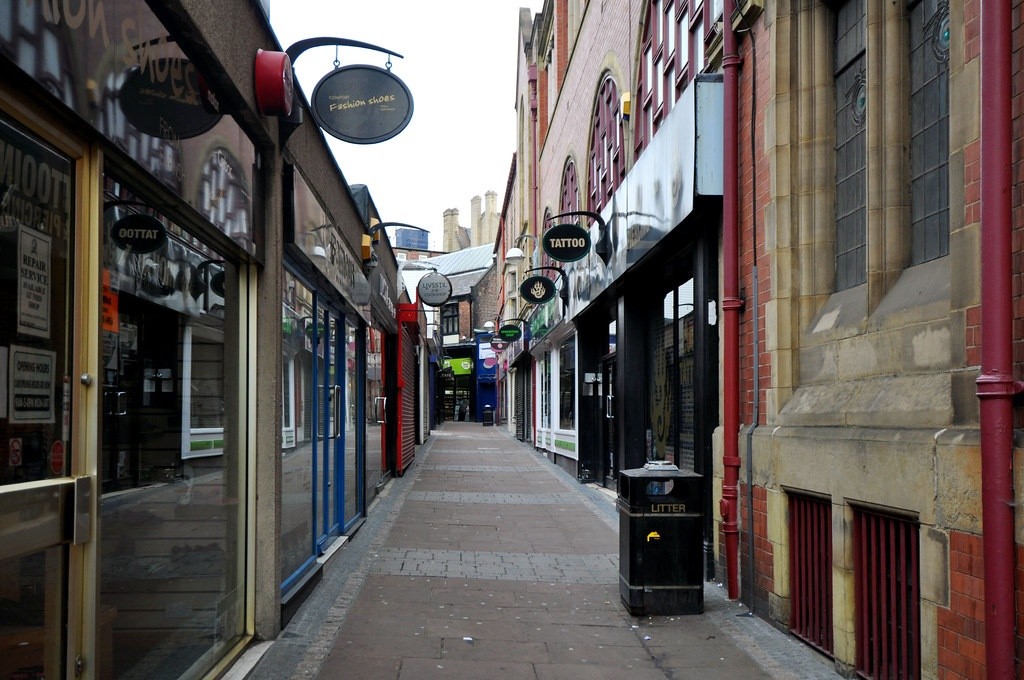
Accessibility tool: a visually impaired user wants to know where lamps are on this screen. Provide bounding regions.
[505,234,536,263]
[484,313,502,327]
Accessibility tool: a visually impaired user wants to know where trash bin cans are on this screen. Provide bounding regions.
[616,460,704,617]
[482,404,493,426]
